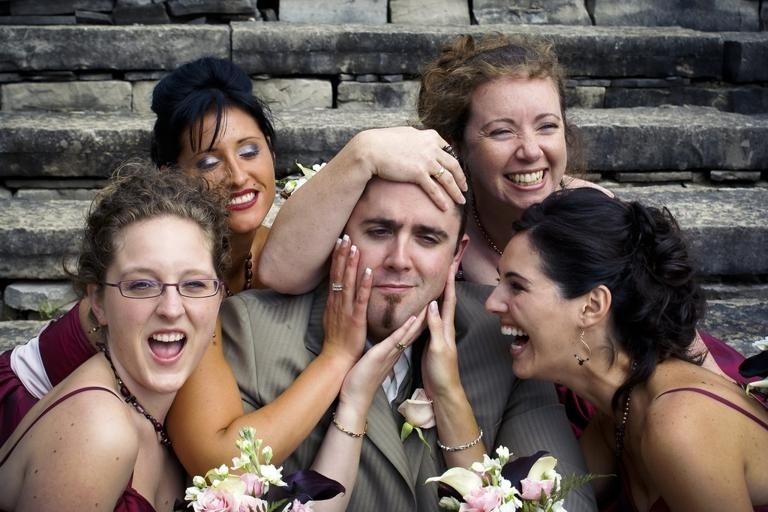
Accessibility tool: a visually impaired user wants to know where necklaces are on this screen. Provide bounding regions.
[613,362,642,447]
[98,342,171,452]
[466,191,505,254]
[216,240,252,299]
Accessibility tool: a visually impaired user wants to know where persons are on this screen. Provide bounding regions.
[218,174,597,509]
[257,36,614,296]
[0,157,231,512]
[0,58,275,445]
[484,188,768,511]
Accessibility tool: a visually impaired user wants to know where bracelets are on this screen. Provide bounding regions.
[436,426,483,452]
[330,410,368,439]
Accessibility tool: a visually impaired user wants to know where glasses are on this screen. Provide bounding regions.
[105,279,224,299]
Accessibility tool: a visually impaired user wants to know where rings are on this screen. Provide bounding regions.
[329,280,344,292]
[396,341,409,351]
[433,170,445,179]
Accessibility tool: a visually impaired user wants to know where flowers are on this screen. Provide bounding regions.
[397,386,440,450]
[425,443,607,512]
[181,424,313,512]
[274,157,327,200]
